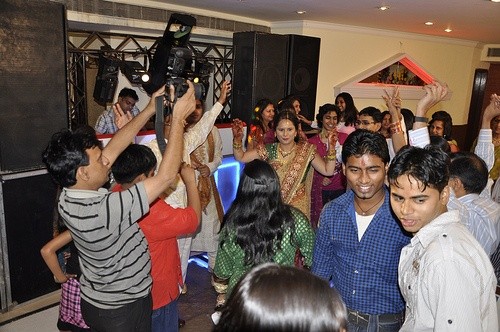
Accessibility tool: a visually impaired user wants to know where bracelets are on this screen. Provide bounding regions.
[325,150,337,162]
[233,141,243,150]
[390,120,402,135]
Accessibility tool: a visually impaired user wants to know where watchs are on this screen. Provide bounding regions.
[412,115,428,124]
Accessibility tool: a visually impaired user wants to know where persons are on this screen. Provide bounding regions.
[209,263,347,332]
[44,80,195,332]
[210,159,315,311]
[309,128,414,332]
[41,80,500,332]
[388,146,500,332]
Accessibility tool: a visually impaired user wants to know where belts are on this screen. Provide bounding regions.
[345,310,404,327]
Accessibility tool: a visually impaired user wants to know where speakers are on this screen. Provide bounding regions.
[287,34,321,142]
[0,0,72,169]
[231,30,289,123]
[0,170,81,309]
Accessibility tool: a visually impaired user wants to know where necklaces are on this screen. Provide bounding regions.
[354,194,384,216]
[278,143,296,157]
